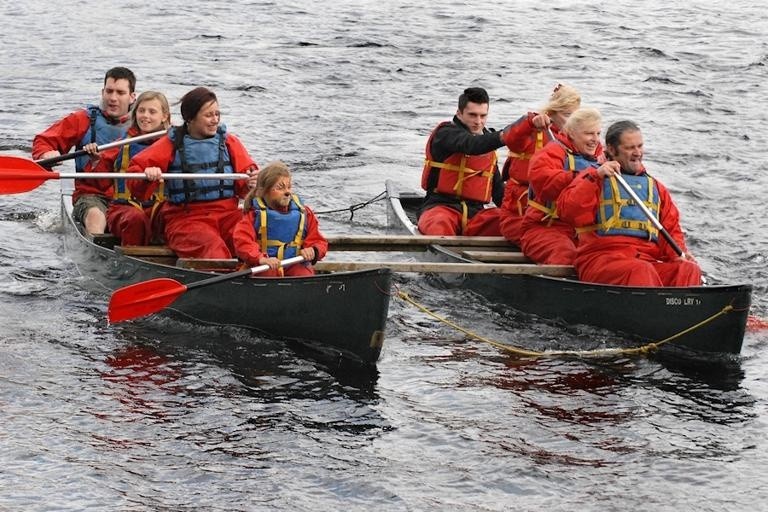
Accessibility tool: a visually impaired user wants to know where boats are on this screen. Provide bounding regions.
[61,189,391,364]
[386,178,753,354]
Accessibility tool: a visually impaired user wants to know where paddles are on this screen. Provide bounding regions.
[0,155,250,194]
[108,255,305,324]
[613,172,768,329]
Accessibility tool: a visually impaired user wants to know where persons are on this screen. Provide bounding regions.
[83,90,171,246]
[31,66,136,235]
[417,88,506,236]
[556,122,703,286]
[520,107,607,266]
[125,87,261,269]
[498,84,581,252]
[232,163,328,277]
